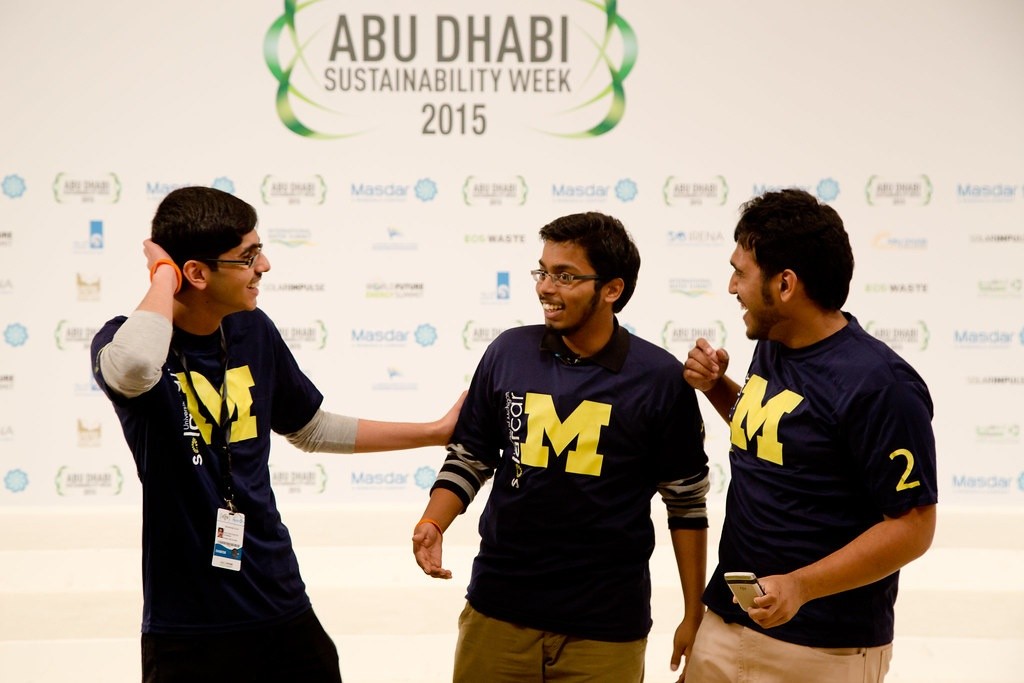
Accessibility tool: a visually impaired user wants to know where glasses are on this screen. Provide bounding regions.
[530,268,597,287]
[206,242,262,267]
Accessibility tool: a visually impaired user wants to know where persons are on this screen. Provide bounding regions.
[683,187,937,683]
[217,527,224,537]
[412,213,710,683]
[85,187,469,683]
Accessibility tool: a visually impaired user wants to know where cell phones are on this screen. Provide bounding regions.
[724,572,766,612]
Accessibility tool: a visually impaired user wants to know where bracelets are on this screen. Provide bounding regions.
[149,258,183,296]
[413,519,445,540]
